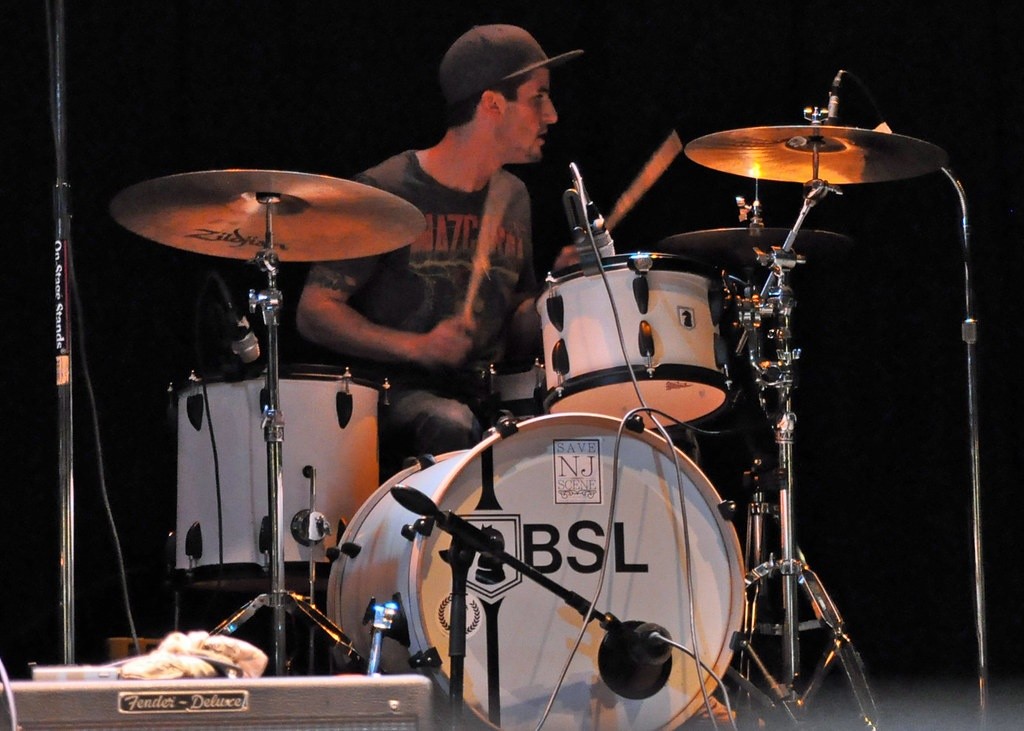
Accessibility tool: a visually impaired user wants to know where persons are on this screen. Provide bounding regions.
[296,23,579,488]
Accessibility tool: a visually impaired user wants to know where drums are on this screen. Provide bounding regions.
[277,353,277,359]
[321,403,756,731]
[165,360,387,590]
[531,248,736,434]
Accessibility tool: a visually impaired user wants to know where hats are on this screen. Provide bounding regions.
[439,24,585,103]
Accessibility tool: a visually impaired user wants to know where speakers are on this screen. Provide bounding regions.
[2,675,499,729]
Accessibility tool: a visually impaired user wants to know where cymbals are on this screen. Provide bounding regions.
[105,165,431,268]
[682,122,955,193]
[653,219,862,275]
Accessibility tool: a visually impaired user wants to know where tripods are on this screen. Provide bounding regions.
[209,202,369,675]
[723,142,880,731]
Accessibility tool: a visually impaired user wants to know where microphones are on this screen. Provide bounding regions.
[568,160,616,257]
[213,273,260,363]
[629,623,674,668]
[827,69,844,125]
